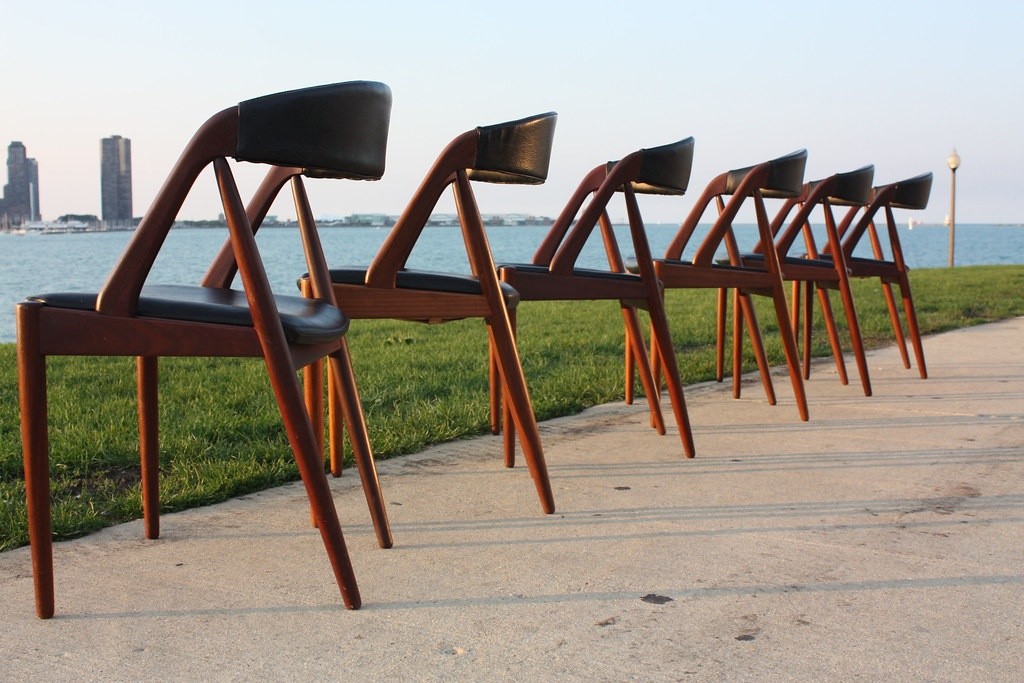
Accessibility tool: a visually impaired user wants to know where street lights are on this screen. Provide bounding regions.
[946,146,961,267]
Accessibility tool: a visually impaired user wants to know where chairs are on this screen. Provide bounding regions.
[490,138,695,468]
[716,165,874,405]
[296,111,559,515]
[625,149,809,428]
[16,80,392,621]
[791,172,933,384]
